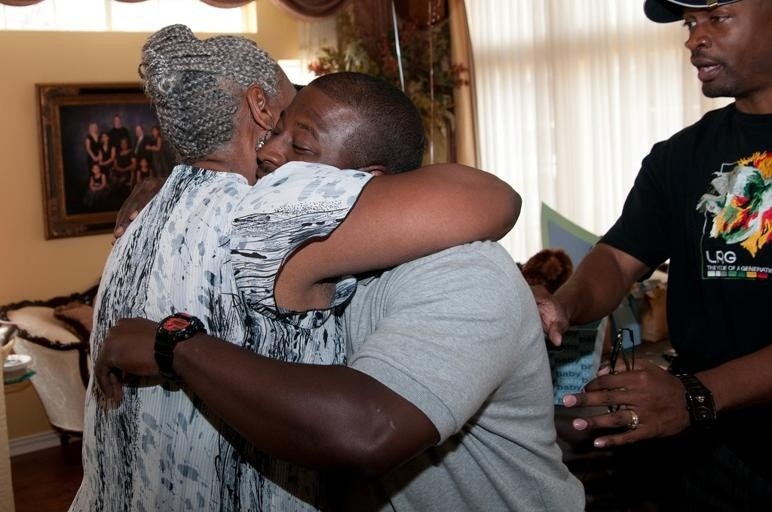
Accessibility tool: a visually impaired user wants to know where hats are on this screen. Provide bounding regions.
[645,0,739,23]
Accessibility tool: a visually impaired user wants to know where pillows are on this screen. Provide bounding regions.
[52,299,96,350]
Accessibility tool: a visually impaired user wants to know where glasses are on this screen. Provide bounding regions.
[601,328,634,435]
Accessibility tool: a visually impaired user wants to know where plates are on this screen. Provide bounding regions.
[3,353,32,372]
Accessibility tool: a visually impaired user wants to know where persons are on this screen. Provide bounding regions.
[83,110,168,212]
[530,2,772,512]
[64,26,523,512]
[95,67,588,512]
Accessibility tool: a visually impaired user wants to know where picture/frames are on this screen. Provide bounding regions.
[33,78,179,242]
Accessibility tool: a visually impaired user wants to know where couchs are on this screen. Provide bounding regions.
[3,290,123,448]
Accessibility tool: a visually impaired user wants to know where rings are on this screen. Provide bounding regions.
[627,409,641,429]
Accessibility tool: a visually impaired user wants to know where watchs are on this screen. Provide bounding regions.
[677,374,717,429]
[153,310,207,392]
